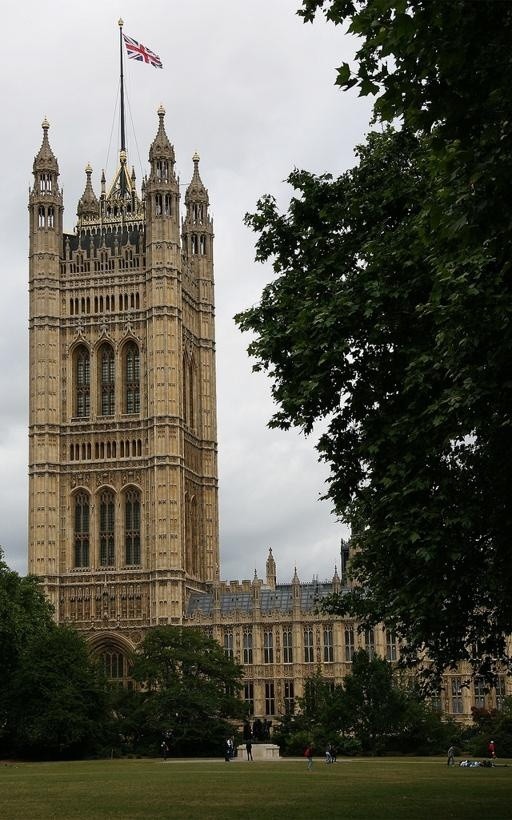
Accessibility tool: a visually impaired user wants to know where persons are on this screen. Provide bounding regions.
[325,742,333,764]
[488,741,497,760]
[253,718,265,742]
[161,741,169,761]
[223,737,232,762]
[261,719,273,744]
[330,746,339,763]
[305,742,317,770]
[246,740,253,762]
[445,746,456,768]
[457,759,496,768]
[244,719,254,740]
[227,735,234,759]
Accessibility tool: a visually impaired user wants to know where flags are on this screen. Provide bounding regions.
[122,33,165,69]
